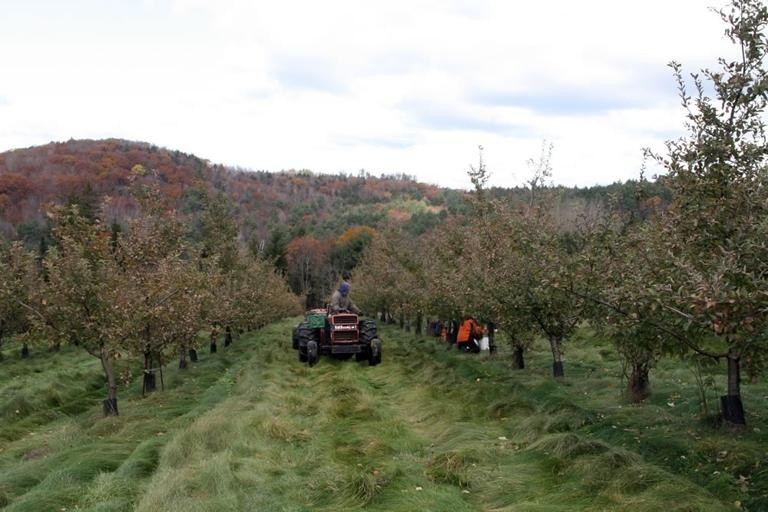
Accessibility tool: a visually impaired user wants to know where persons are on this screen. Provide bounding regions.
[331,282,364,317]
[430,316,489,354]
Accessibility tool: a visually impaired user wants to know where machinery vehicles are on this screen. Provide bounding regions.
[292,305,381,368]
